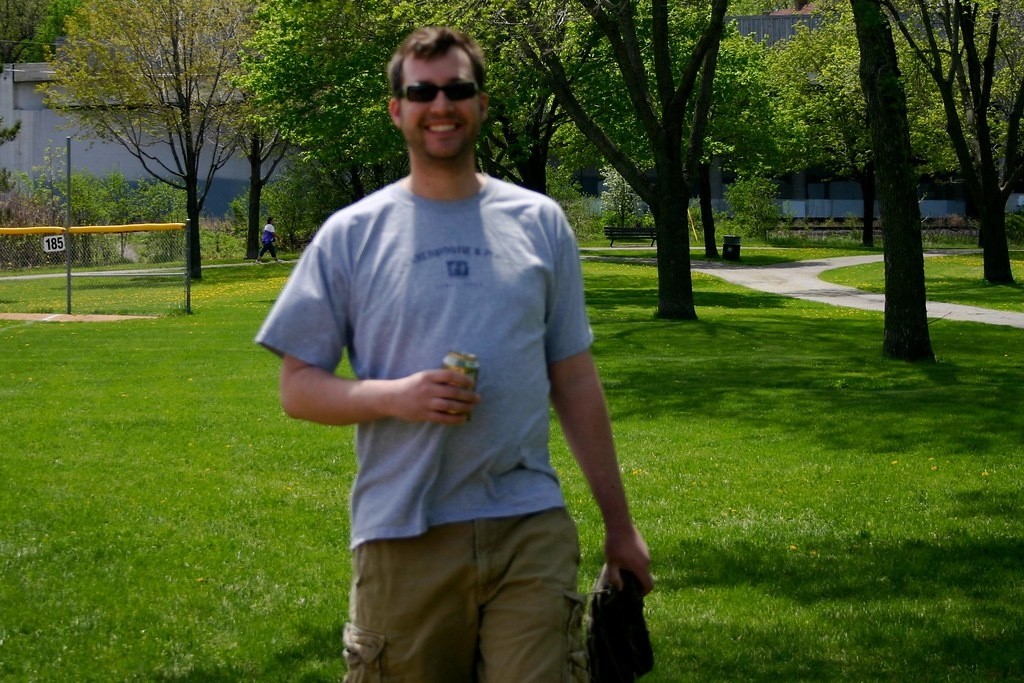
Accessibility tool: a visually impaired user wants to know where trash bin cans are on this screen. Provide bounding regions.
[722,235,741,261]
[979,228,984,247]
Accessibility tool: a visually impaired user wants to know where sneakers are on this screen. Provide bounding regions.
[255,260,263,264]
[274,260,283,263]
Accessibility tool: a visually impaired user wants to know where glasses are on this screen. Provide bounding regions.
[399,81,479,103]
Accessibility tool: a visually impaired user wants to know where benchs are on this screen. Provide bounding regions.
[603,226,657,247]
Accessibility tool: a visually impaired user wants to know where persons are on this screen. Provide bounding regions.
[256,217,283,265]
[255,26,655,682]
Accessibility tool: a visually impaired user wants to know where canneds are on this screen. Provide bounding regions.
[440,351,479,421]
